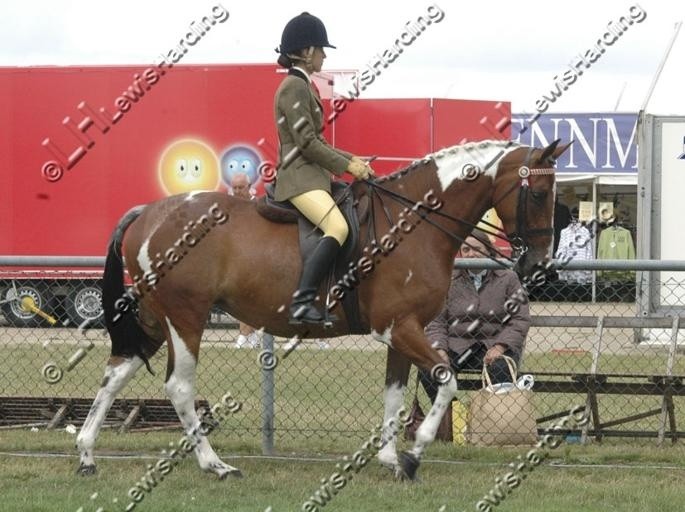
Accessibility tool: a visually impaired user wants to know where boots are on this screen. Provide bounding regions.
[288,236,339,324]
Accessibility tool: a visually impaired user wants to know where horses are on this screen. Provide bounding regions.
[73,137,577,485]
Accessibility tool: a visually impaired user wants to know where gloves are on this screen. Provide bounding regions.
[346,156,374,181]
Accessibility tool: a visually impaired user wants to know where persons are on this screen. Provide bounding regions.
[273,11,377,327]
[418,227,532,443]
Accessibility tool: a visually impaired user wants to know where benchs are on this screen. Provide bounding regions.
[403,315,685,446]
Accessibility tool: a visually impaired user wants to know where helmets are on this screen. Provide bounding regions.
[280,13,336,52]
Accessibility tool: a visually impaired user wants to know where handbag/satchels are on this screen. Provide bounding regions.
[466,389,540,447]
[405,398,456,440]
